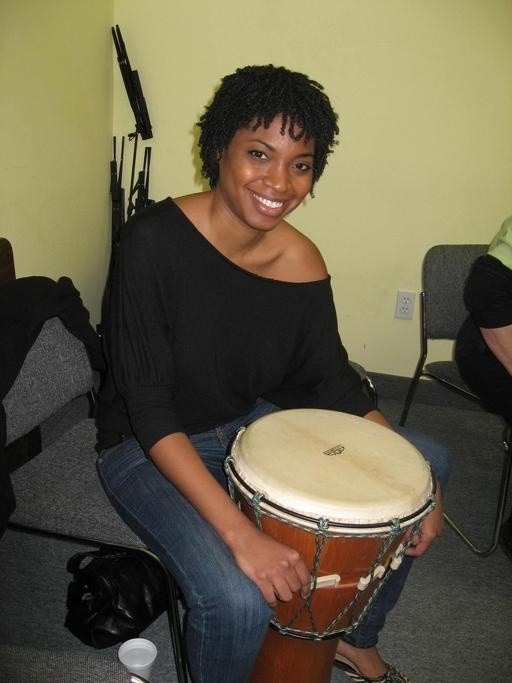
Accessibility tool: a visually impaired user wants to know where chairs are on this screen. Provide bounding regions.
[400,242,512,555]
[1,277,190,682]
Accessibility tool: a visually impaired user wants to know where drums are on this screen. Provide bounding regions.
[224,409,436,682]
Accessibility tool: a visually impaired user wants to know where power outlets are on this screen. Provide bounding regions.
[393,290,417,320]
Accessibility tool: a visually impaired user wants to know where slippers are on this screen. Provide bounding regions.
[333,651,408,682]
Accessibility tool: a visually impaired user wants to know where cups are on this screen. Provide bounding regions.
[117,637,158,683]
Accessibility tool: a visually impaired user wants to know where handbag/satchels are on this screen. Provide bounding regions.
[64,546,190,681]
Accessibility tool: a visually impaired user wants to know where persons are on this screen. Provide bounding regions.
[87,58,445,682]
[453,214,512,430]
